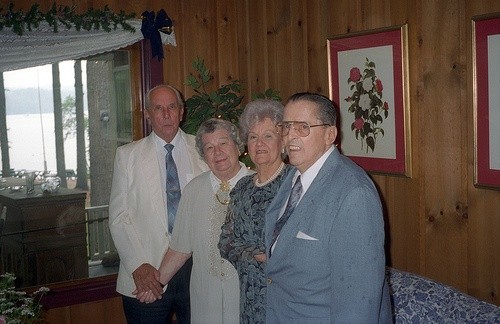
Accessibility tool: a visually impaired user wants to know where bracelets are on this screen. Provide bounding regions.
[160,283,164,288]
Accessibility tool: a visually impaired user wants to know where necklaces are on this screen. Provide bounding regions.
[256,161,284,187]
[215,193,229,205]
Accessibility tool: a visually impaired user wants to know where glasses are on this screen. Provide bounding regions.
[274,120,331,137]
[151,105,181,113]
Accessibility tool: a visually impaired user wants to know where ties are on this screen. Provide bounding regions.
[270,175,303,249]
[163,144,182,234]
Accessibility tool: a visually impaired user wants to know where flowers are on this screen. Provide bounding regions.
[0,272,50,324]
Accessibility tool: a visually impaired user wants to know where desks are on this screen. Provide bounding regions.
[0,177,89,291]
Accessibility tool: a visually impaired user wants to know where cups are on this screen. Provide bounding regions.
[25,170,35,194]
[0,178,6,191]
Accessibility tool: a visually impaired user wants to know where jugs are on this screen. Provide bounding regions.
[41,173,61,193]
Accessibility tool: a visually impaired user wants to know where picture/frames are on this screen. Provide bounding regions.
[325,23,414,180]
[471,11,500,191]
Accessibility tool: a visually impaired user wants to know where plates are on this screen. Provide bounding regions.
[8,186,23,191]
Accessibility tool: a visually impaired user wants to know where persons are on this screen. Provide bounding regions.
[265,92,394,324]
[108,85,210,324]
[218,99,292,324]
[132,119,258,324]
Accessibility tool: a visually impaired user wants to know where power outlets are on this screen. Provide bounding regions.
[100,109,111,123]
